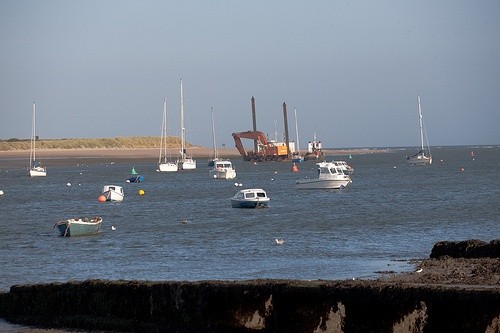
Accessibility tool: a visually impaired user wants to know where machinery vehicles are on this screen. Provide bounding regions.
[232,130,288,162]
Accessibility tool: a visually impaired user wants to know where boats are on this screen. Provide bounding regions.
[231,189,270,208]
[295,166,352,189]
[53,216,103,236]
[27,102,47,177]
[175,78,196,169]
[406,95,432,165]
[157,98,178,171]
[208,107,230,167]
[316,160,354,175]
[101,185,124,202]
[292,108,305,163]
[127,175,144,183]
[209,159,236,179]
